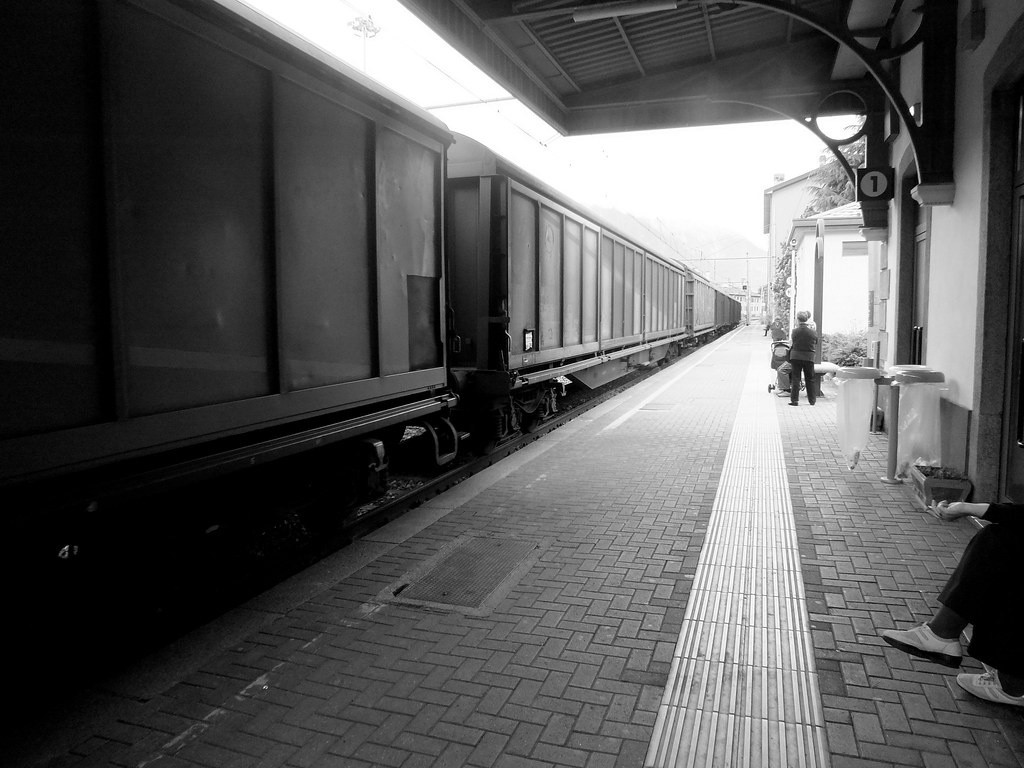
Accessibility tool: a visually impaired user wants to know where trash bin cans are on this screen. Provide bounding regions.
[896,370,946,479]
[836,366,879,470]
[885,366,930,440]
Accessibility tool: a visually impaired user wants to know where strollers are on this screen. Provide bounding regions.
[767,340,805,396]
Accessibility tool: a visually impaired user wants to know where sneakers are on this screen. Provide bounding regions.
[882,621,962,669]
[956,670,1024,707]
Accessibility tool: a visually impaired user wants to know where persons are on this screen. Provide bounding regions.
[771,316,785,343]
[880,499,1024,709]
[788,311,819,405]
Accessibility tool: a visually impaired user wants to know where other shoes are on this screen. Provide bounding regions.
[777,391,791,397]
[788,402,798,406]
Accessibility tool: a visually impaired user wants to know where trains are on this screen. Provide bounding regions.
[0,0,744,712]
